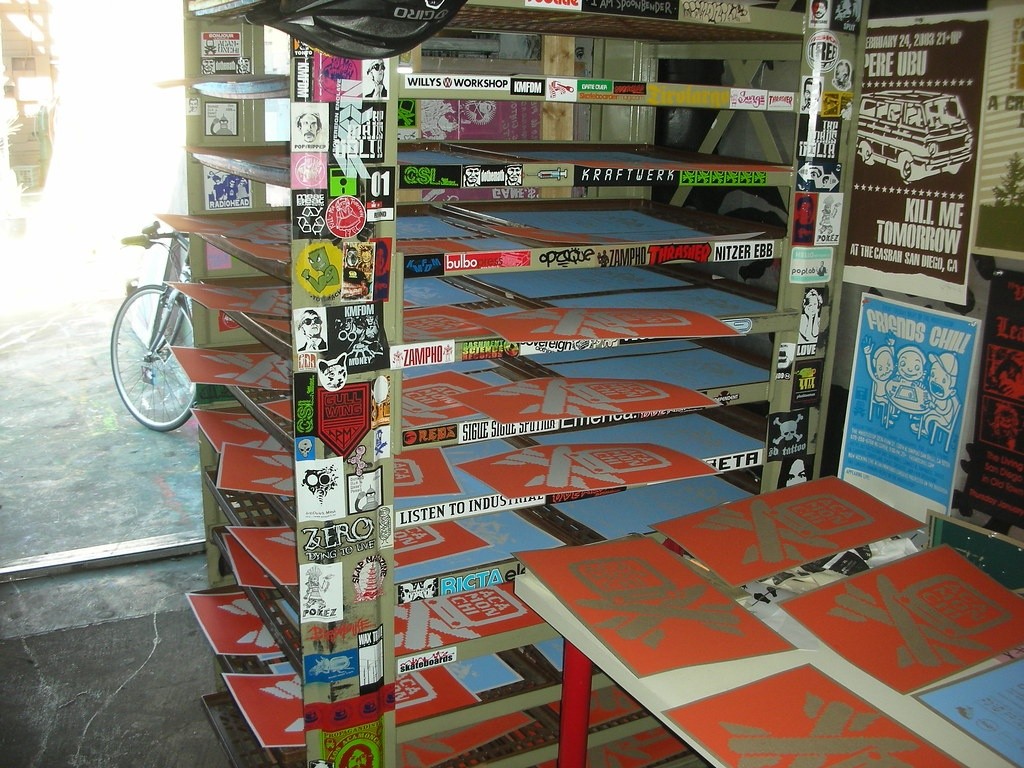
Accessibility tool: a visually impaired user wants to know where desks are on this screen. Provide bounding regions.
[515,528,1024,768]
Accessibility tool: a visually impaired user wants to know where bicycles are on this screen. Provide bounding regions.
[110,221,197,432]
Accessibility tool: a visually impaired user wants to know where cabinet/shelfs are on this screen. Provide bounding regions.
[178,0,806,768]
